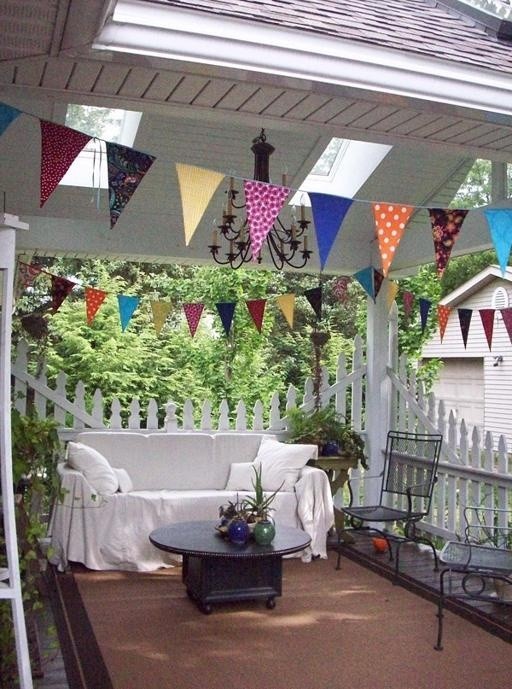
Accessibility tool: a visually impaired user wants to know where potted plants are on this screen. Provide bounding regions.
[282,403,354,456]
[226,461,285,547]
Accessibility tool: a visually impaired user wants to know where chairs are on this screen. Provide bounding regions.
[334,429,444,587]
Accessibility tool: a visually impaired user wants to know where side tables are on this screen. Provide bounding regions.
[307,455,359,544]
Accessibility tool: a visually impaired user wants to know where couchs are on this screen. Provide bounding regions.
[55,430,325,562]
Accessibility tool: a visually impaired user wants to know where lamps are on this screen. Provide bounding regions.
[207,128,314,272]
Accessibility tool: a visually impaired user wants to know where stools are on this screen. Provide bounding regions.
[433,506,512,652]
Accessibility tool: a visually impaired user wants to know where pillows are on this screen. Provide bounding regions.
[225,437,319,493]
[64,441,134,496]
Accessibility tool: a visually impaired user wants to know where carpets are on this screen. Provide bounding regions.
[41,540,512,689]
